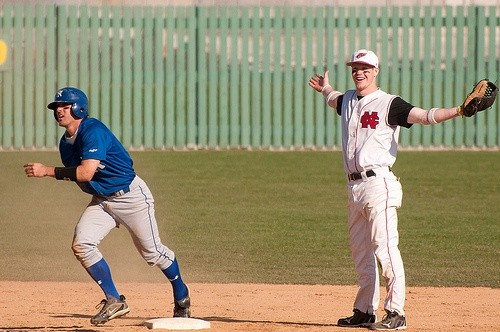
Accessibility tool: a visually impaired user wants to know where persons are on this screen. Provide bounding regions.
[23,88,190,326]
[308,49,499,331]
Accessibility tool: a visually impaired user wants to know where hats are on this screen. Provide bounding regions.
[346,49,378,69]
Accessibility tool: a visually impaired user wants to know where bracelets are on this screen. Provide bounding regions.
[457,105,462,116]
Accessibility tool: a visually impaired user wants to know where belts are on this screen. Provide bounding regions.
[348,166,391,180]
[107,186,130,196]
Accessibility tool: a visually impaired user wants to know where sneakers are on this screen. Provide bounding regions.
[90,295,130,324]
[338,308,375,327]
[170,285,190,318]
[372,311,406,330]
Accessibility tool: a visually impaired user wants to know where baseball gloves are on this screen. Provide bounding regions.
[461,79,499,117]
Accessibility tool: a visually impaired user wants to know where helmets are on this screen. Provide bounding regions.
[47,87,89,118]
